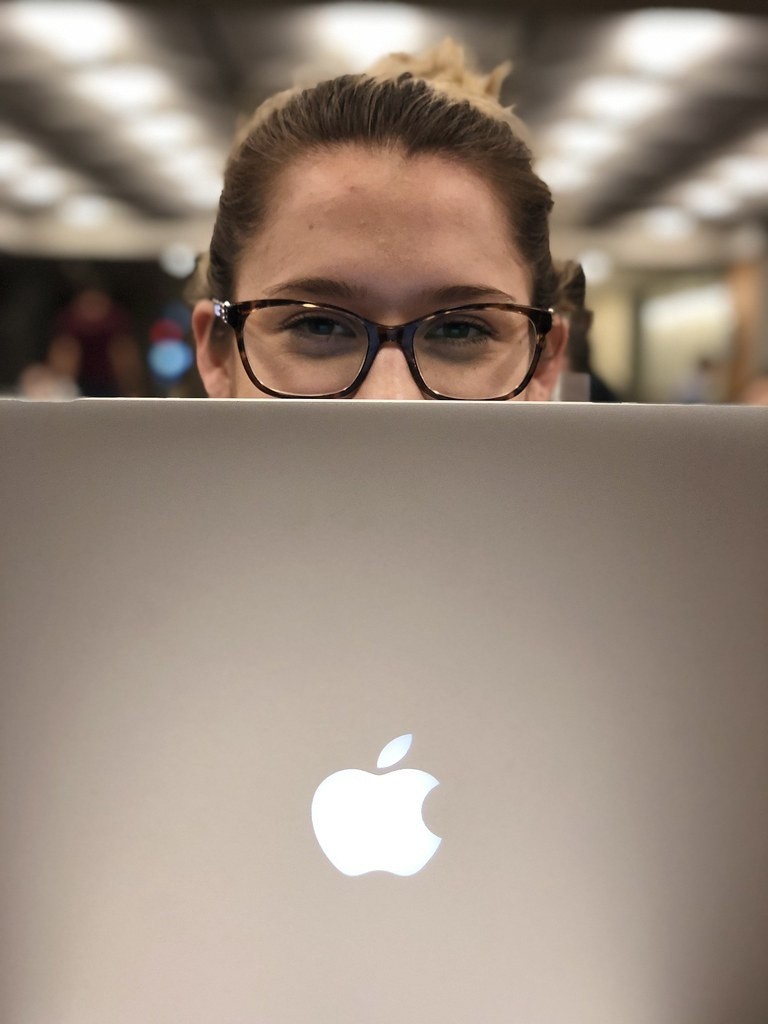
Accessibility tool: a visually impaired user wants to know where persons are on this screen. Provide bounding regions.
[190,39,572,401]
[18,283,139,397]
[555,262,617,401]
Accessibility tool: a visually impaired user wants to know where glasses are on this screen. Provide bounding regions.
[209,298,552,401]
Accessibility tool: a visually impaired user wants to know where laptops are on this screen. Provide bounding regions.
[0,398,768,1024]
